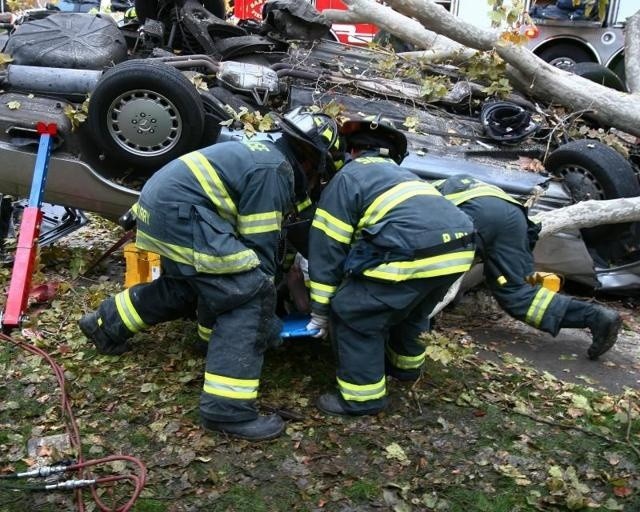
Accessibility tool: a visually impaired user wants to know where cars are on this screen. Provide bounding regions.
[0,4,640,309]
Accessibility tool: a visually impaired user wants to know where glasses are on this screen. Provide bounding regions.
[284,106,324,149]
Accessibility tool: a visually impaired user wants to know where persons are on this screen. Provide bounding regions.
[429,174,622,361]
[74,105,343,442]
[301,119,476,417]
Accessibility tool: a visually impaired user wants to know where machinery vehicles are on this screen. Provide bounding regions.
[228,0,639,90]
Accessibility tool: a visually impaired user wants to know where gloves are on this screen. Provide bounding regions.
[307,313,328,339]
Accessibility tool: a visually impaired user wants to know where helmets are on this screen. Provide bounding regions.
[343,112,407,166]
[269,110,337,157]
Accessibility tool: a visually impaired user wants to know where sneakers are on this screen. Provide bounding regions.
[203,411,284,440]
[587,304,620,357]
[318,394,349,414]
[78,314,129,355]
